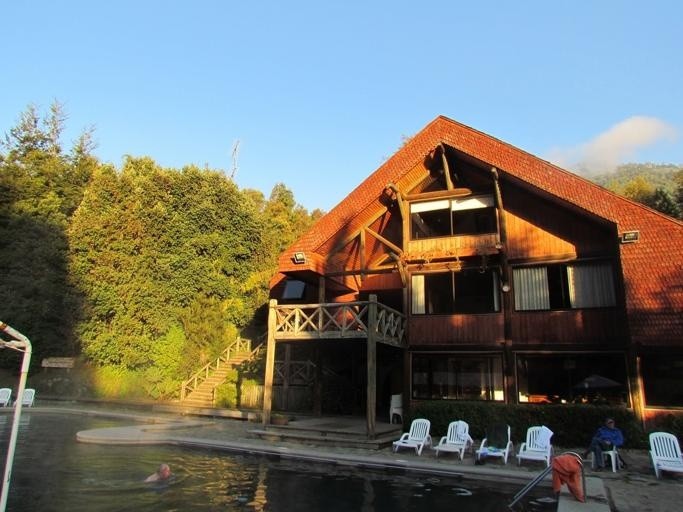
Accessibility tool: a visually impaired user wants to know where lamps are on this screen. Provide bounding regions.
[292,251,306,264]
[620,229,639,244]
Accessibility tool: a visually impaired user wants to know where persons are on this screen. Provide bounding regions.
[581,418,624,472]
[144,463,171,483]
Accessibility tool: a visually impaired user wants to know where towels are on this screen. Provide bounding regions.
[535,425,553,449]
[456,421,473,445]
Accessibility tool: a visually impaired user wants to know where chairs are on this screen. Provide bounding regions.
[515,426,554,468]
[647,432,682,480]
[431,420,469,463]
[474,425,512,465]
[591,444,621,474]
[391,419,431,457]
